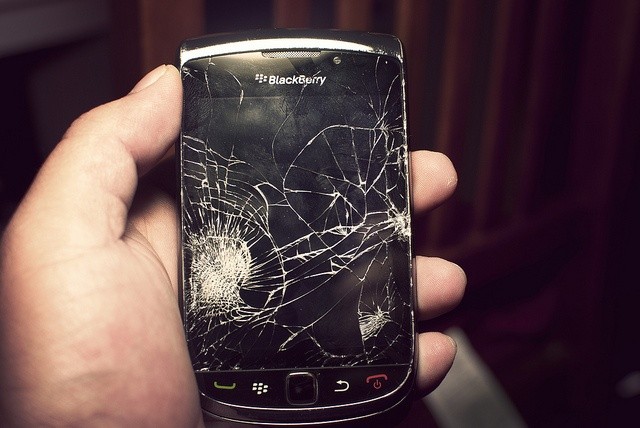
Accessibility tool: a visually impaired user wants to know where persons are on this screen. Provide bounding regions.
[1,63,468,428]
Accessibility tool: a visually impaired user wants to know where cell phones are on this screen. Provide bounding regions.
[180,29,419,427]
